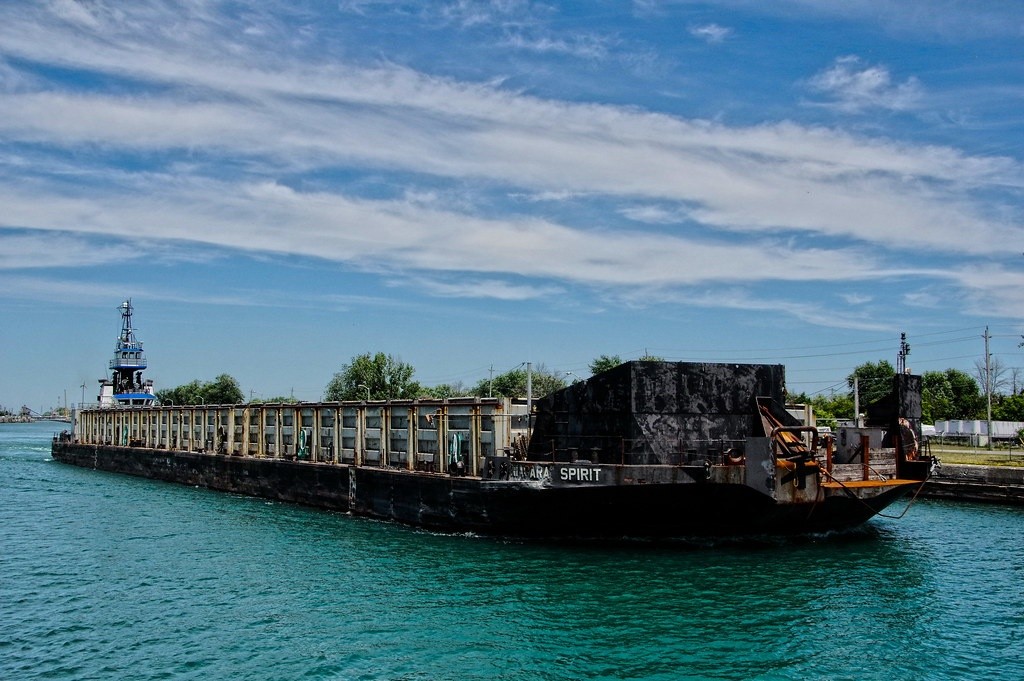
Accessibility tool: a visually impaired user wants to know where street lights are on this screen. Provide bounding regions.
[358,384,370,401]
[194,395,205,404]
[165,398,174,406]
